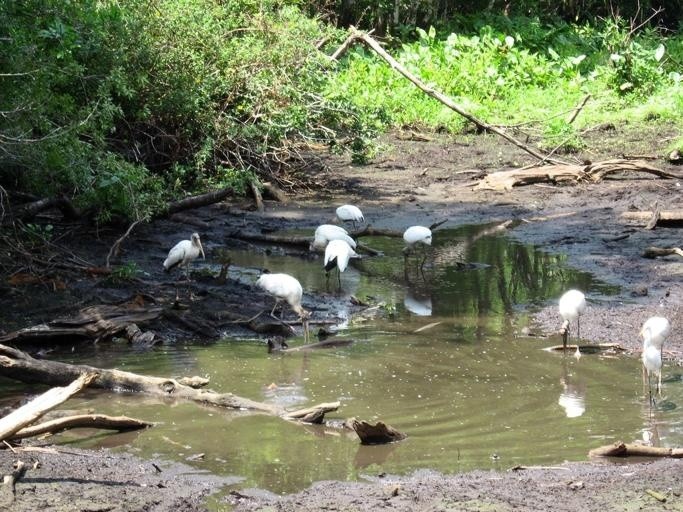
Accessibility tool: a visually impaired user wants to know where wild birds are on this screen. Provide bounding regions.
[639,316,669,398]
[330,205,364,232]
[559,288,586,356]
[322,239,361,291]
[557,357,585,419]
[254,273,310,344]
[308,223,356,254]
[403,282,433,317]
[401,225,432,283]
[161,232,205,302]
